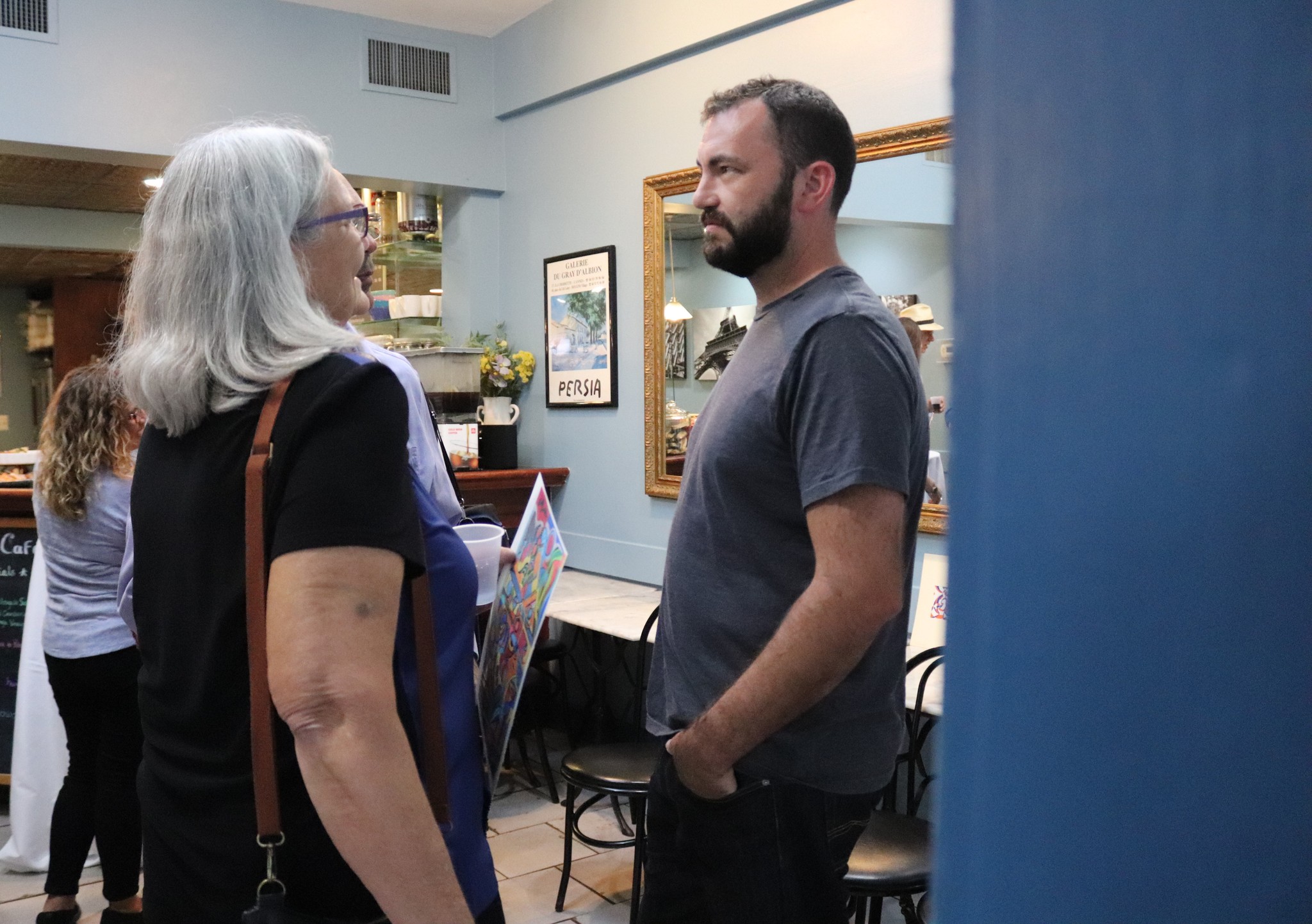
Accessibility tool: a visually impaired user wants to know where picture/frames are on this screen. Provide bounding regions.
[543,245,618,409]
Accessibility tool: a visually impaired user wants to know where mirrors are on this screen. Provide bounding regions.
[643,113,948,537]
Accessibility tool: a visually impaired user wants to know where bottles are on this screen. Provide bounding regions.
[361,188,439,243]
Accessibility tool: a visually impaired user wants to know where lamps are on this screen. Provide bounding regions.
[665,214,693,322]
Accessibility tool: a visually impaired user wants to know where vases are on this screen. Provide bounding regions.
[477,396,519,425]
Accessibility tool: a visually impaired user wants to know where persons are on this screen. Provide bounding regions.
[29,362,160,924]
[104,123,513,924]
[636,75,927,924]
[895,301,946,509]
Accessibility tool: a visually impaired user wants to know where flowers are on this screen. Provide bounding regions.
[463,321,535,397]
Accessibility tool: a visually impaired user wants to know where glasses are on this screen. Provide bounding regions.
[349,213,383,240]
[292,206,369,239]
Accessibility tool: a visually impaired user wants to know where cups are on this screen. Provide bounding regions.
[450,522,506,608]
[389,294,442,321]
[930,396,941,414]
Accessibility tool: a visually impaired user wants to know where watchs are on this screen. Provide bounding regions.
[926,483,939,496]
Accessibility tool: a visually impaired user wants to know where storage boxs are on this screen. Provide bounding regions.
[398,346,484,393]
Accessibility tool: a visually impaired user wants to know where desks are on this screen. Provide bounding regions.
[512,571,945,803]
[454,467,570,528]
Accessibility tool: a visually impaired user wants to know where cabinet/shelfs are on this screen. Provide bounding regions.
[352,240,441,338]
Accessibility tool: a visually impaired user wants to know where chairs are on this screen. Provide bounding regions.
[555,604,660,924]
[843,645,945,924]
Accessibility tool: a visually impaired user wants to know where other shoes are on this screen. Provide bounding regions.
[99,897,144,924]
[36,902,82,924]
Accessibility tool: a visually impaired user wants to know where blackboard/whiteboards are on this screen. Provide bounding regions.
[0,516,39,785]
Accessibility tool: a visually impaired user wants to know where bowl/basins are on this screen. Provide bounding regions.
[369,289,396,321]
